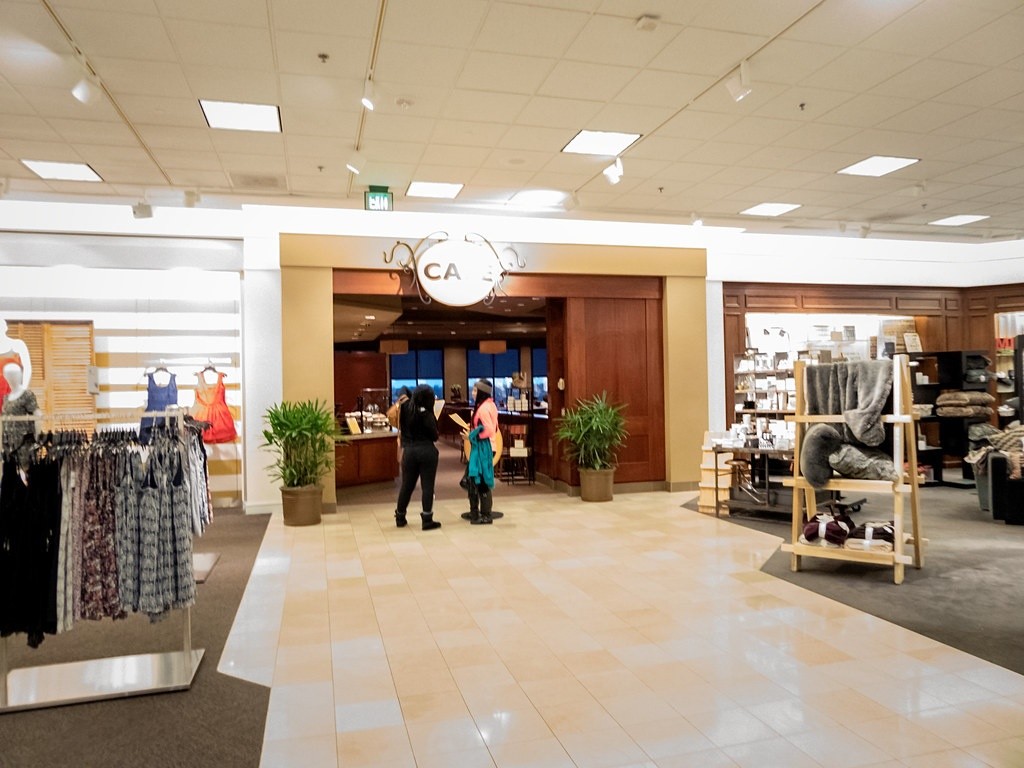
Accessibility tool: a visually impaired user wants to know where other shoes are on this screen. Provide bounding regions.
[461,512,479,519]
[470,516,493,525]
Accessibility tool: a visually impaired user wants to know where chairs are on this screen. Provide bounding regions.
[459,423,537,487]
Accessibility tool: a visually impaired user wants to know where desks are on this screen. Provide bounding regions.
[712,443,835,519]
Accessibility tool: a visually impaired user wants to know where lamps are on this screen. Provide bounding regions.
[347,152,366,176]
[362,73,383,112]
[858,224,871,238]
[689,213,702,226]
[603,157,624,186]
[72,76,104,105]
[725,59,753,102]
[130,198,153,219]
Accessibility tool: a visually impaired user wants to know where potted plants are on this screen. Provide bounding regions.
[257,398,353,524]
[555,391,628,500]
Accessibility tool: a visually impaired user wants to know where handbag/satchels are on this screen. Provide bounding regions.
[459,468,469,489]
[396,405,404,462]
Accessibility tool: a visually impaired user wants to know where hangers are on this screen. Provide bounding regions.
[1,404,206,464]
[145,359,175,381]
[196,357,227,379]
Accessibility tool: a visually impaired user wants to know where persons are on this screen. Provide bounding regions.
[0,318,32,415]
[0,364,47,450]
[461,379,498,524]
[395,384,441,530]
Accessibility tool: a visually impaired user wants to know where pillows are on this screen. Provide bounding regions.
[935,406,994,417]
[937,392,996,407]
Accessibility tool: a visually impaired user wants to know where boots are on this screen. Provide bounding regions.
[421,511,441,530]
[394,509,407,527]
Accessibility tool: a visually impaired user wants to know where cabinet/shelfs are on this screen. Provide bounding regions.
[775,354,923,587]
[735,369,794,483]
[890,349,994,490]
